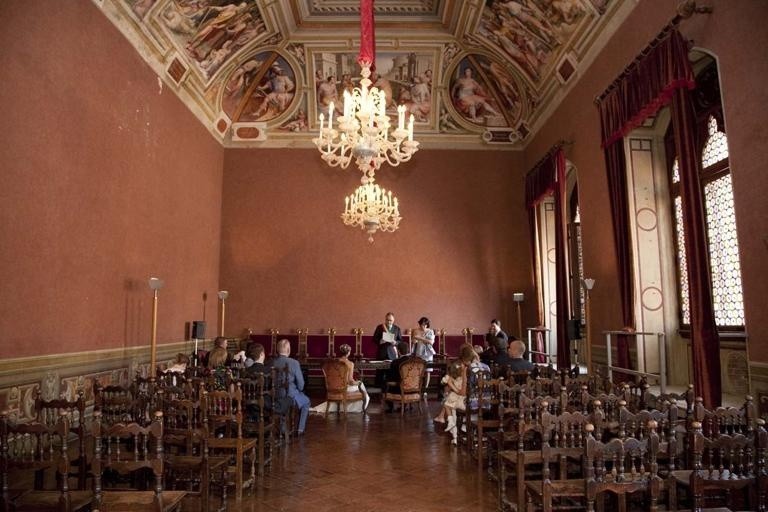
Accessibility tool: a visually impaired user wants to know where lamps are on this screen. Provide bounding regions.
[311,0,421,246]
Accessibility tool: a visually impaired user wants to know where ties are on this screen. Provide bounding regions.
[389,325,390,328]
[492,331,497,335]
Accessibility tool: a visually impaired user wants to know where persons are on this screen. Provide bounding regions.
[202,336,231,370]
[160,1,265,77]
[477,55,518,107]
[491,319,508,353]
[481,338,513,366]
[474,345,484,356]
[249,66,294,117]
[193,350,207,369]
[243,344,272,448]
[309,344,370,413]
[433,362,470,432]
[498,341,534,407]
[160,353,190,387]
[316,69,432,121]
[373,312,402,393]
[231,60,263,95]
[451,68,500,123]
[280,108,305,130]
[231,339,254,380]
[267,339,311,436]
[507,334,517,344]
[482,0,585,80]
[448,343,490,444]
[478,333,498,359]
[408,317,436,396]
[385,342,412,414]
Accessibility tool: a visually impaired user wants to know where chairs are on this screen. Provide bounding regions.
[668,418,768,512]
[380,355,429,419]
[93,366,223,422]
[320,358,369,420]
[156,389,234,512]
[527,368,755,419]
[522,400,605,512]
[0,408,93,512]
[199,381,258,502]
[34,389,96,491]
[583,420,660,512]
[484,376,542,482]
[93,411,187,512]
[225,369,276,476]
[606,398,678,512]
[497,386,568,512]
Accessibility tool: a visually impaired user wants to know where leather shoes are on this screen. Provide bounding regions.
[451,438,457,444]
[434,416,445,423]
[443,419,456,434]
[385,407,394,414]
[396,406,408,412]
[296,430,303,435]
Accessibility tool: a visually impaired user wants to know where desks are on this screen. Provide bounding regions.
[354,359,448,402]
